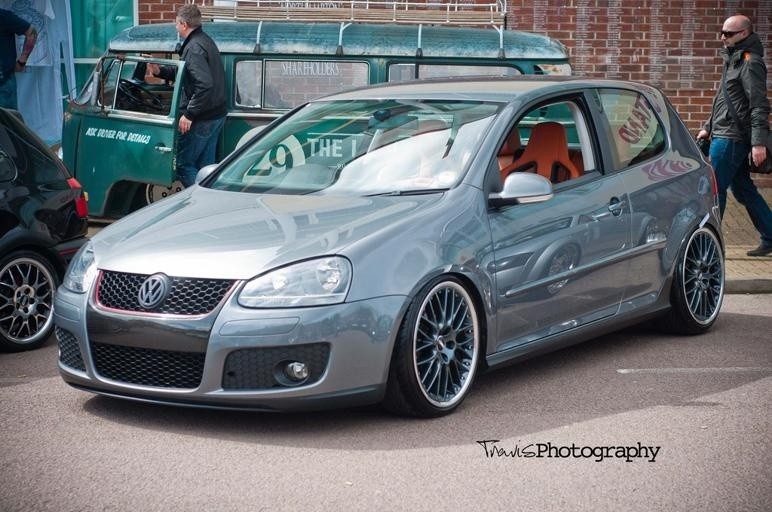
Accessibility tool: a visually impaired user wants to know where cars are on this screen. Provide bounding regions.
[54,77,725,412]
[0,108,90,349]
[467,203,657,308]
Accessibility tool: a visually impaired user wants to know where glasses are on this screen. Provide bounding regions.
[721,30,744,38]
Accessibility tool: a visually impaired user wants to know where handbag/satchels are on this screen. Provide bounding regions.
[751,130,772,174]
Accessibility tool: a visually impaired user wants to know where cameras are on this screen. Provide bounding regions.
[696,138,710,156]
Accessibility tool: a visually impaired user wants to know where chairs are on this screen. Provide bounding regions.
[500,122,579,185]
[494,127,525,172]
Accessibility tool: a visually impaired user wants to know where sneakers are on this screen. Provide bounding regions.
[746,244,772,256]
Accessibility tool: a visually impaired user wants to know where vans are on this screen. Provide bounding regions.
[63,22,579,224]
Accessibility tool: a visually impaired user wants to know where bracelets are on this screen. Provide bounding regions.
[17,59,26,66]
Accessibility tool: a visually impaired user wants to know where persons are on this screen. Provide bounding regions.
[141,3,229,187]
[0,8,38,110]
[696,14,772,256]
